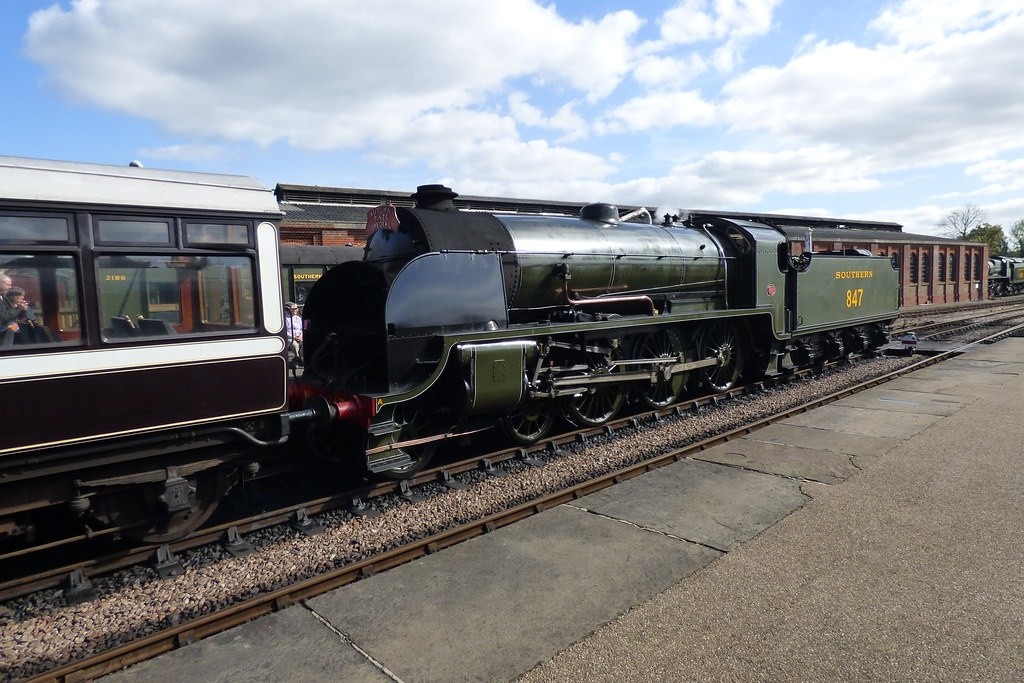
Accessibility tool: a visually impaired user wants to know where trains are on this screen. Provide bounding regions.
[0,156,903,587]
[983,253,1024,300]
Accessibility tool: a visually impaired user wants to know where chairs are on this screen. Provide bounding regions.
[16,320,62,343]
[135,315,176,334]
[109,315,137,334]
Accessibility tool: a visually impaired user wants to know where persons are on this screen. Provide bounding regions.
[0,274,37,336]
